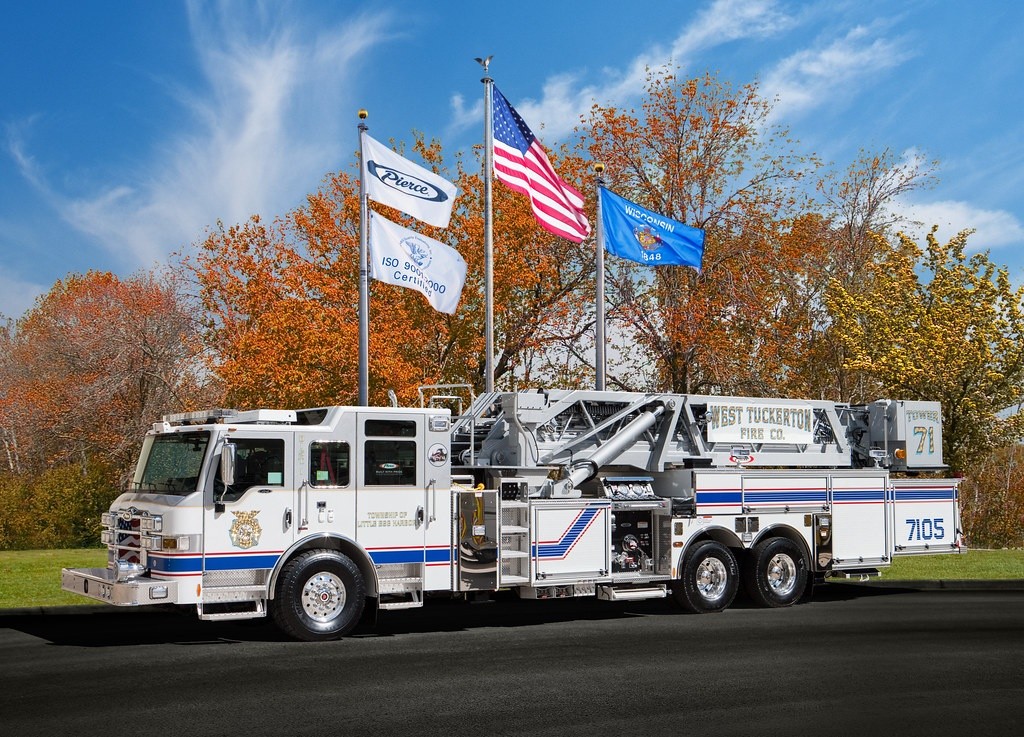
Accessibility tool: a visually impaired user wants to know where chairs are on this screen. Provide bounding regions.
[259,455,282,476]
[215,452,245,476]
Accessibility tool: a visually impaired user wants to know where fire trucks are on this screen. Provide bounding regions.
[59,377,970,641]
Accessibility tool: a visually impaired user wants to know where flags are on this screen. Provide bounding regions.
[601,186,705,268]
[362,131,459,229]
[490,84,592,246]
[369,209,470,316]
